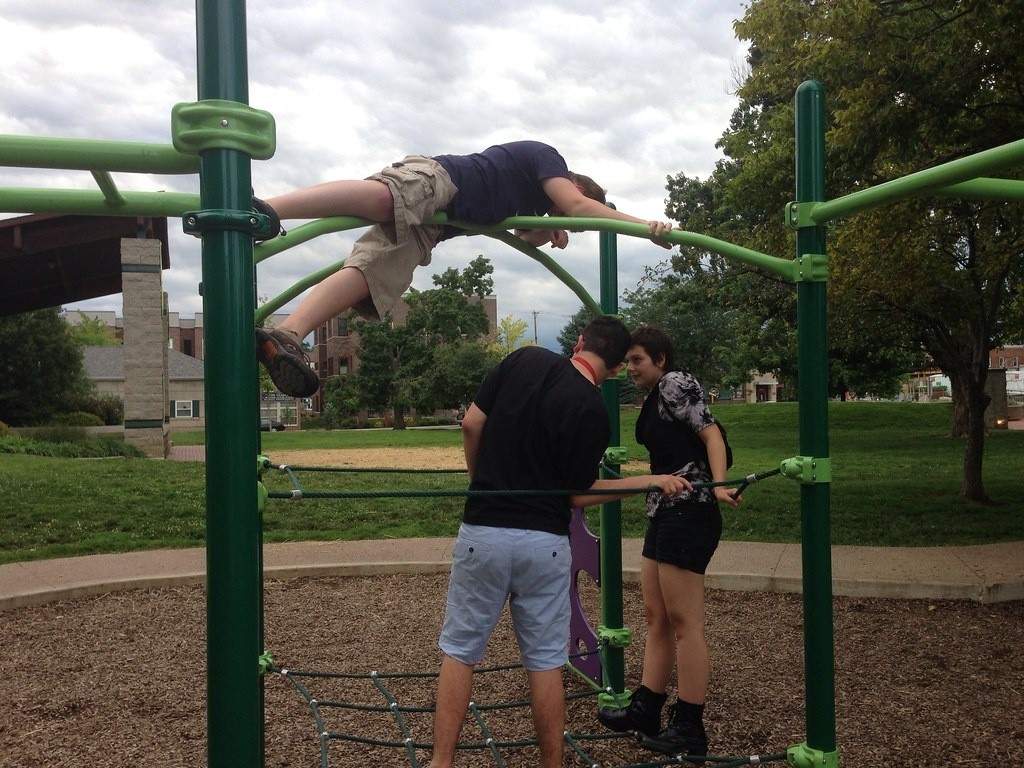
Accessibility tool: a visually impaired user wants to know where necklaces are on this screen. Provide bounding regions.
[573,357,598,385]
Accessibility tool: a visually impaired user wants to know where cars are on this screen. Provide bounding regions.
[260,419,286,432]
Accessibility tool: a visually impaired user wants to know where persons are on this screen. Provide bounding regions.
[252,141,684,398]
[599,327,742,763]
[430,316,632,768]
